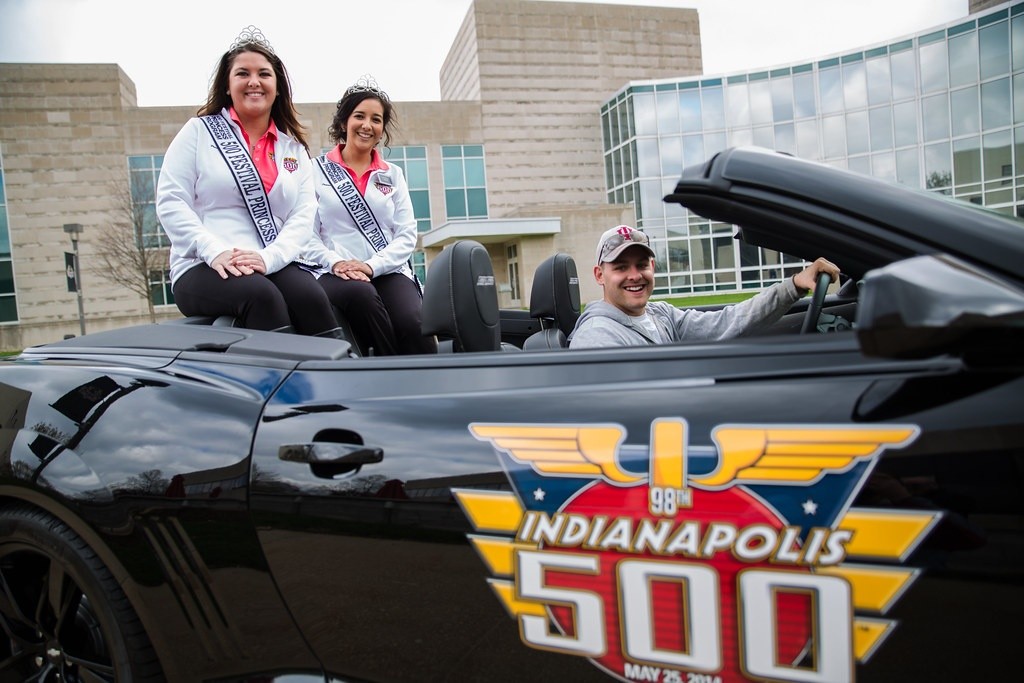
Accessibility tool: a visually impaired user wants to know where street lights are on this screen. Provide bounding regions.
[62,223,86,336]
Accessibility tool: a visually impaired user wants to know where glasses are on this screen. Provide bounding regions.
[598,231,649,265]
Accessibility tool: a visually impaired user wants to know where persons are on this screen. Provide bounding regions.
[567,225,840,348]
[300,75,440,358]
[154,25,358,361]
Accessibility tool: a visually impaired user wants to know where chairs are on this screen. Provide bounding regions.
[420,238,522,352]
[523,252,582,352]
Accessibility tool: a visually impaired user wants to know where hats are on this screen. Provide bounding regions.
[596,225,656,266]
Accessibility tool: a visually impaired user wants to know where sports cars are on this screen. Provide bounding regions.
[1,143,1024,683]
